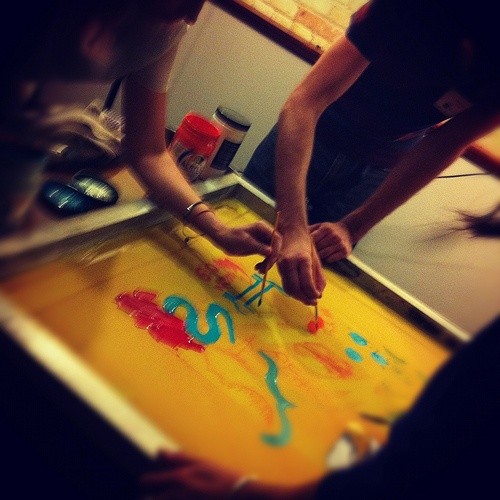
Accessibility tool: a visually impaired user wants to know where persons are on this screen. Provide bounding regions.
[243,0,500,306]
[156,309,499,500]
[0,1,284,274]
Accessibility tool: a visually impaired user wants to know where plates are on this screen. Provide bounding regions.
[38,181,91,215]
[71,173,118,207]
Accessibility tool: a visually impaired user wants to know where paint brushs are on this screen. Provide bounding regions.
[255,212,281,308]
[311,239,324,334]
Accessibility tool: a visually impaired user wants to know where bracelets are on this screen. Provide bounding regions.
[182,200,216,240]
[224,473,258,500]
